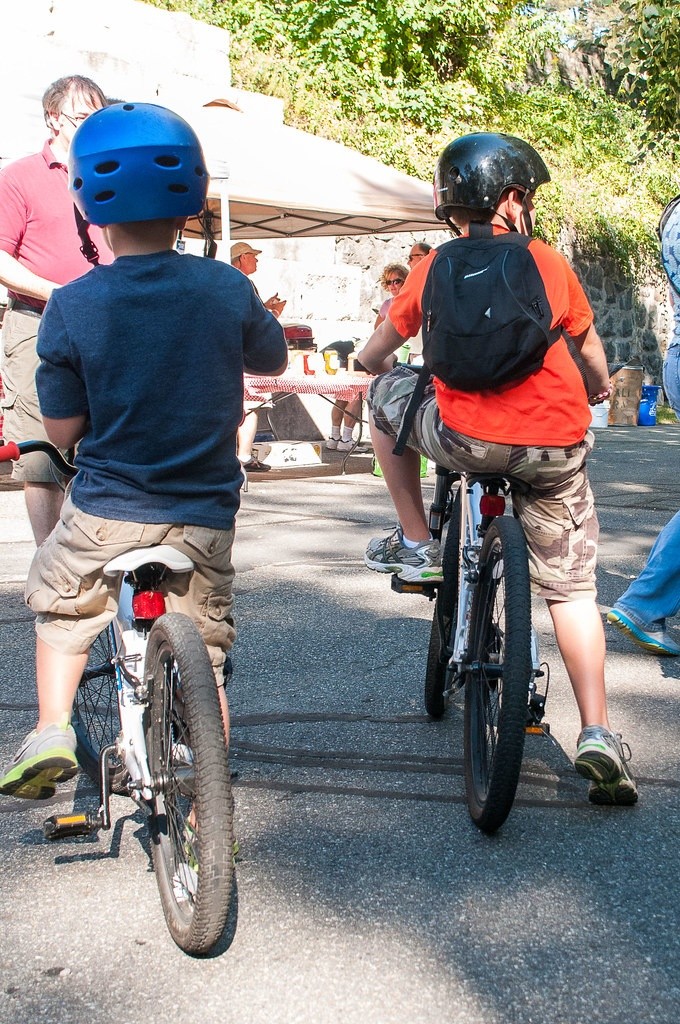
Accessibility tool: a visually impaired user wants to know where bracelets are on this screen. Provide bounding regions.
[272,309,279,316]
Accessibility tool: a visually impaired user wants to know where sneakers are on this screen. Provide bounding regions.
[180,815,238,895]
[607,608,680,655]
[575,723,638,807]
[363,522,445,580]
[325,436,369,452]
[0,723,79,800]
[242,457,271,473]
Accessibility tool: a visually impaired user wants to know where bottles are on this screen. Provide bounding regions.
[324,347,338,375]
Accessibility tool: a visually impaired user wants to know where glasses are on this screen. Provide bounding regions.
[409,254,423,261]
[61,110,86,123]
[386,278,402,285]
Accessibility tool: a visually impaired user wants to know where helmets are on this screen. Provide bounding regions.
[67,102,208,225]
[433,133,551,221]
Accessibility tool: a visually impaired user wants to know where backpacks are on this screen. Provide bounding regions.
[391,219,563,457]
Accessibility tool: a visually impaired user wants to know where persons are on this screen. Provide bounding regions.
[2,100,289,867]
[322,337,369,452]
[351,132,638,810]
[406,243,432,365]
[230,242,287,472]
[0,74,116,549]
[373,263,409,331]
[605,192,680,655]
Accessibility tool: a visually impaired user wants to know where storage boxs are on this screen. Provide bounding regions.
[284,324,314,350]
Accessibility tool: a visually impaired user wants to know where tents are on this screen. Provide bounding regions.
[172,101,464,269]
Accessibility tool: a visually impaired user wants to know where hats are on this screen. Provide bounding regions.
[230,242,262,261]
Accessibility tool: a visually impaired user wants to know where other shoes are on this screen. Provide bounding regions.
[172,743,193,766]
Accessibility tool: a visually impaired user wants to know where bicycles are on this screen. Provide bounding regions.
[0,440,230,955]
[354,358,549,834]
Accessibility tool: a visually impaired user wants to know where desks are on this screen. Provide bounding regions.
[235,369,376,492]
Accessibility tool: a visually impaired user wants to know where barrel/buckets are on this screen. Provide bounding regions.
[609,366,643,426]
[638,386,662,426]
[588,399,610,428]
